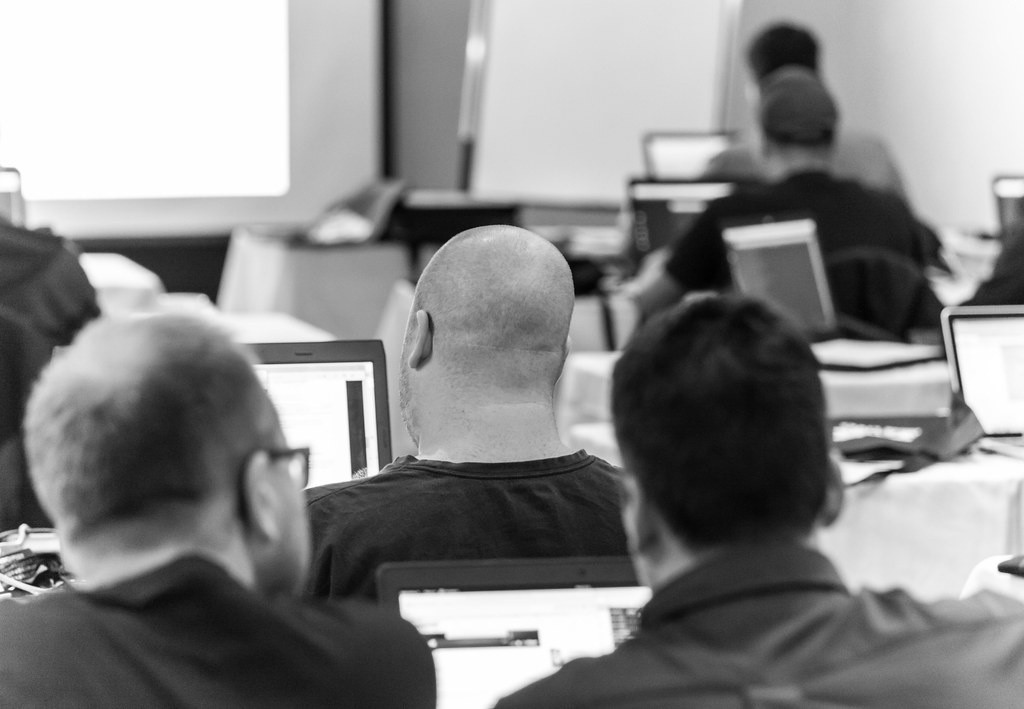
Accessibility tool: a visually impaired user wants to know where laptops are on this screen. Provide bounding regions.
[0,129,1024,709]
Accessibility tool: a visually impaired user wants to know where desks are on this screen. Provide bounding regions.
[812,434,1024,605]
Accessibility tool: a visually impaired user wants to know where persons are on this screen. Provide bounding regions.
[699,26,905,201]
[495,297,1024,708]
[627,75,926,339]
[300,225,642,602]
[0,311,437,709]
[1,219,100,528]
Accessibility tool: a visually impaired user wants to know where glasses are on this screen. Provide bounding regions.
[270,446,310,487]
